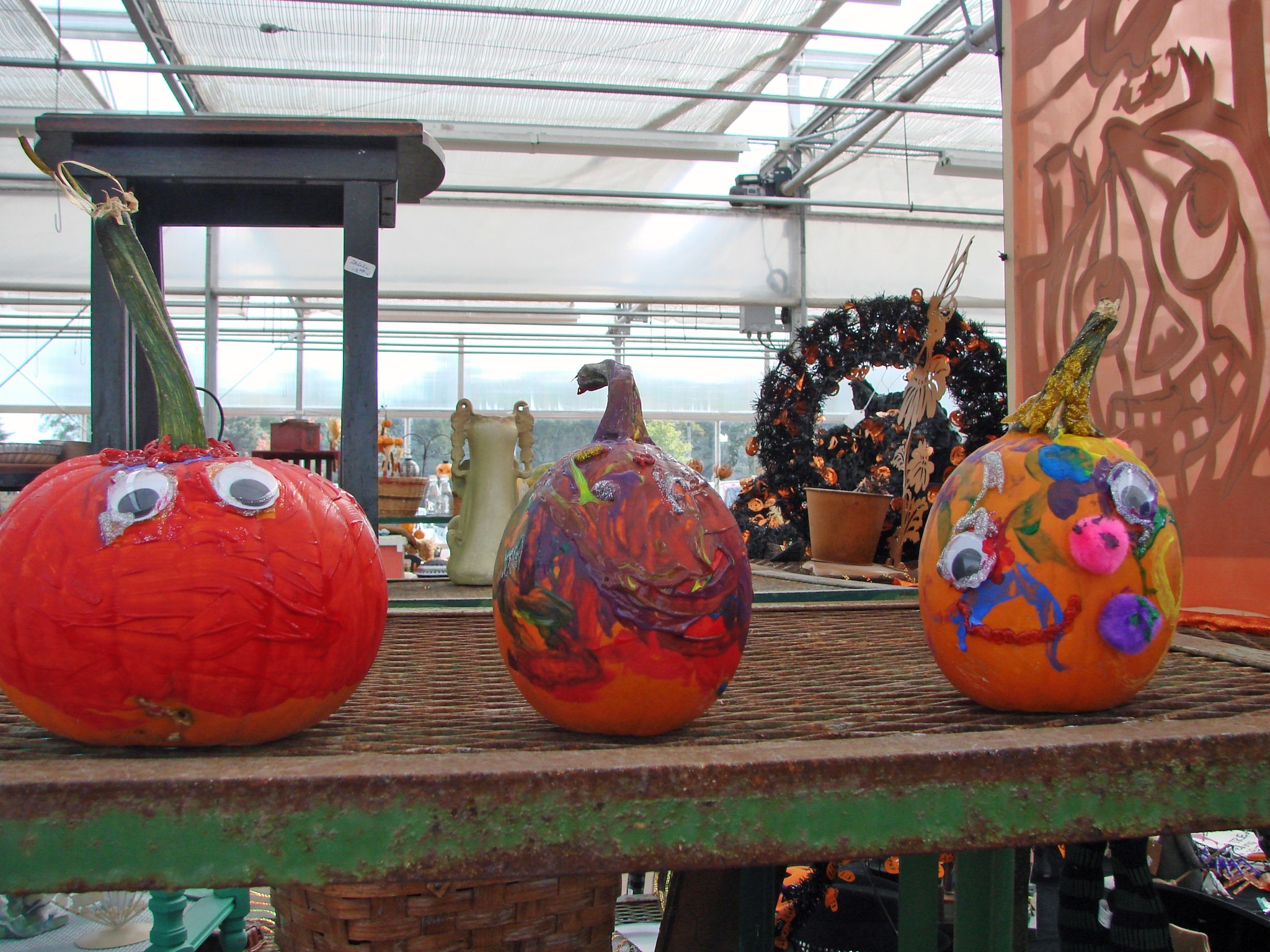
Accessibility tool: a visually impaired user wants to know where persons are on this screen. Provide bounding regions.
[1155,832,1203,894]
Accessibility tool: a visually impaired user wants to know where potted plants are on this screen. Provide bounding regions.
[802,417,903,565]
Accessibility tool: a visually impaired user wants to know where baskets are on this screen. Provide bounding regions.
[377,449,428,517]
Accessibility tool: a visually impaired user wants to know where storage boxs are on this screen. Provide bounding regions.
[379,535,408,578]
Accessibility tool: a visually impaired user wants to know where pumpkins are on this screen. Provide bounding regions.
[490,359,753,738]
[917,297,1184,713]
[0,184,390,748]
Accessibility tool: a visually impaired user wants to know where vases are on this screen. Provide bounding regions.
[379,476,429,517]
